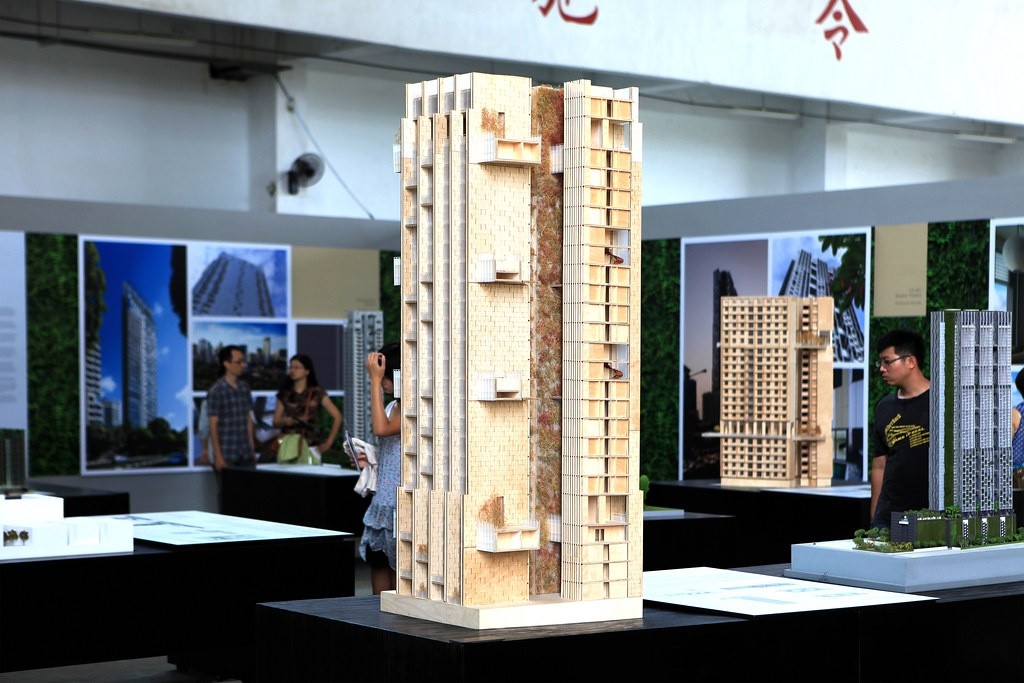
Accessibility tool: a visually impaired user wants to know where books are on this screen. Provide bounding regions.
[344,420,361,476]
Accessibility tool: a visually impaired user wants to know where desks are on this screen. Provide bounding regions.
[219,458,362,534]
[645,479,882,569]
[1,510,355,673]
[644,505,736,572]
[253,563,1024,683]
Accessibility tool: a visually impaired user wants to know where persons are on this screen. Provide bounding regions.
[350,342,401,596]
[199,400,256,487]
[207,345,257,517]
[870,329,930,537]
[273,354,343,465]
[1011,367,1024,469]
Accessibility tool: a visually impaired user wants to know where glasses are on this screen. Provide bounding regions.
[874,355,911,368]
[231,357,244,365]
[289,364,305,371]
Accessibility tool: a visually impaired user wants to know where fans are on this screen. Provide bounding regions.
[288,153,324,194]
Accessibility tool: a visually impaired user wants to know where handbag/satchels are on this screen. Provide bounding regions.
[276,432,315,465]
